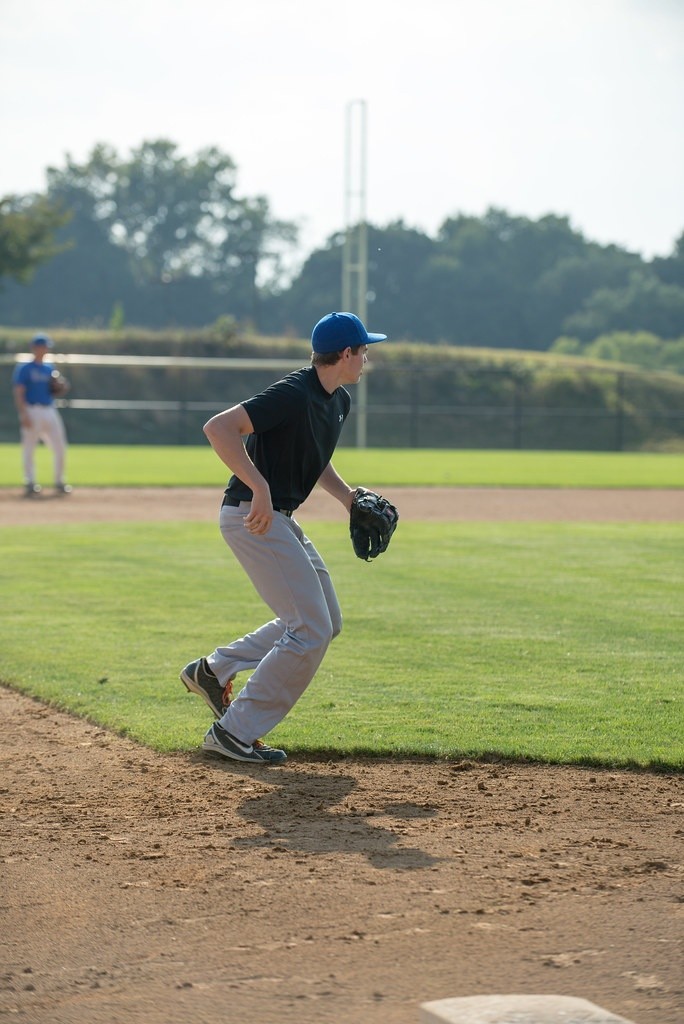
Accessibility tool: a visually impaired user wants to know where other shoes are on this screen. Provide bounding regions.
[57,488,69,495]
[27,490,40,498]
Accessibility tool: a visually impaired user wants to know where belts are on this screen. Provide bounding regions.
[27,401,46,406]
[223,495,292,517]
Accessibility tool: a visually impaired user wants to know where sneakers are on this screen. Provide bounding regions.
[180,656,234,719]
[202,718,287,762]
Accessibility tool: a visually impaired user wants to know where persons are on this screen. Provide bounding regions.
[180,311,399,764]
[14,336,70,497]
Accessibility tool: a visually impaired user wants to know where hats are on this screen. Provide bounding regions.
[311,311,387,352]
[34,336,48,344]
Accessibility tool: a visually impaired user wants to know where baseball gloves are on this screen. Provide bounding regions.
[352,485,399,561]
[49,372,70,397]
